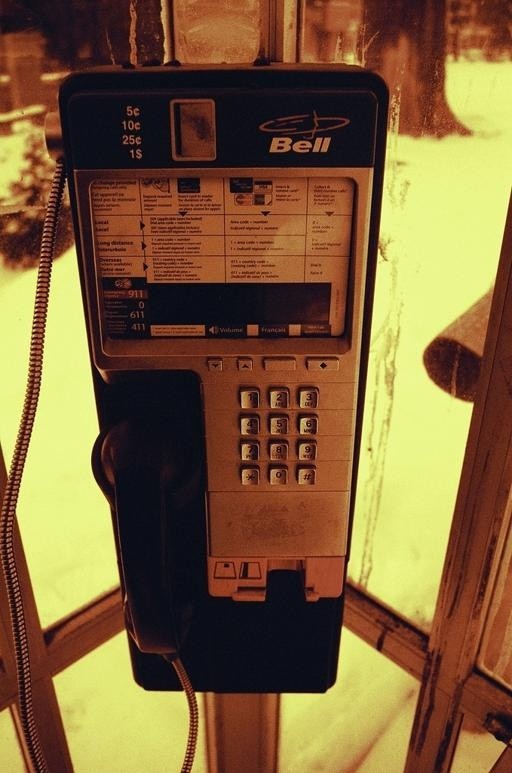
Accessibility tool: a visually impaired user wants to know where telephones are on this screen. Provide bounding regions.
[59,63,393,695]
